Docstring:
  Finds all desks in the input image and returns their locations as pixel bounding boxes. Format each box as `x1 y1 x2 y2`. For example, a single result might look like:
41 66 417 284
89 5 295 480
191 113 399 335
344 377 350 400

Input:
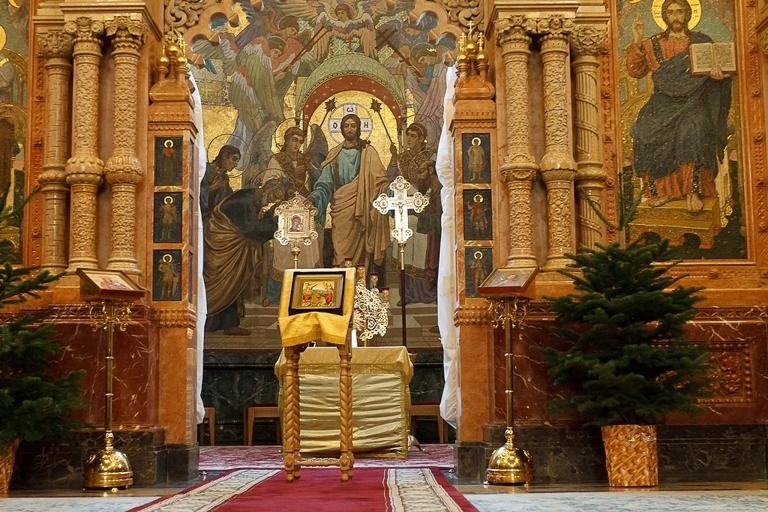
276 346 409 460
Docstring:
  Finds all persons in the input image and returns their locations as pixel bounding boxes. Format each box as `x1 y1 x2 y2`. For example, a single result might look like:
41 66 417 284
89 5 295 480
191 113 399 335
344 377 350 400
204 178 287 336
289 215 301 232
200 145 243 219
159 195 177 241
158 138 178 186
470 250 488 298
307 114 391 291
467 137 488 182
491 272 518 286
263 127 310 198
158 253 178 298
386 122 443 306
626 0 733 215
467 194 489 238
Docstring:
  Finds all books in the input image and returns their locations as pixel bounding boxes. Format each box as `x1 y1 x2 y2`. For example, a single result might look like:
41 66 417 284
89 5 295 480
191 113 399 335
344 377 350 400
688 42 738 76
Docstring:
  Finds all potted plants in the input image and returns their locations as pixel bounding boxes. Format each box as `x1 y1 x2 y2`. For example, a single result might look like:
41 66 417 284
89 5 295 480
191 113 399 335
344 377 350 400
0 180 85 498
535 172 715 487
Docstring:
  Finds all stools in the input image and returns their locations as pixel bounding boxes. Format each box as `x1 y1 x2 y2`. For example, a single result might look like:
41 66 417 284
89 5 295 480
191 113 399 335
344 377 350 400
198 402 449 447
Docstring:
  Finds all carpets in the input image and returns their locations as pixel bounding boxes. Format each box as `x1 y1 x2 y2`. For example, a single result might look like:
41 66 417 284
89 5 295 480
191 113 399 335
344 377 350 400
129 468 477 512
197 447 455 469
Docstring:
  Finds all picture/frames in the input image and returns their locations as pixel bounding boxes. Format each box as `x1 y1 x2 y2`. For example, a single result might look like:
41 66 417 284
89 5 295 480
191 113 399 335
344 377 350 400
77 267 147 298
479 266 537 294
288 271 346 315
